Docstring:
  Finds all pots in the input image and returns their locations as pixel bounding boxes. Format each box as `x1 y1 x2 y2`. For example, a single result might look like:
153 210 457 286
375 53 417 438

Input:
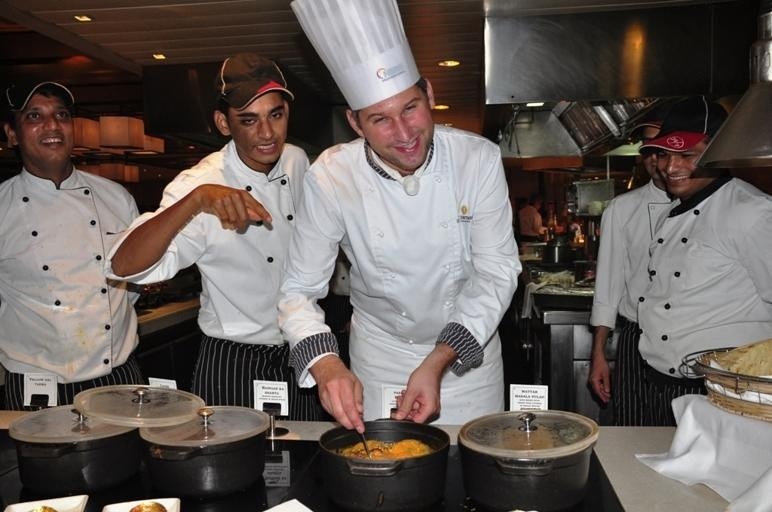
547 244 572 265
315 412 598 511
9 385 268 499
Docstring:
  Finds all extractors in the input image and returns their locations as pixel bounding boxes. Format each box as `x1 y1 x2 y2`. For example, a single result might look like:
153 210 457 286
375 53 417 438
483 36 695 158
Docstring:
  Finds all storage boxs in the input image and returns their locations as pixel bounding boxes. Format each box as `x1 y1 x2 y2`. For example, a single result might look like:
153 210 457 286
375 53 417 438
70 116 164 182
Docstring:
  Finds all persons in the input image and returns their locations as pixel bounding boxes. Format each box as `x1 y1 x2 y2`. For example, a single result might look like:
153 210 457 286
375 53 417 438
586 98 676 426
517 192 549 243
1 73 147 410
637 93 770 427
274 74 522 434
101 52 328 422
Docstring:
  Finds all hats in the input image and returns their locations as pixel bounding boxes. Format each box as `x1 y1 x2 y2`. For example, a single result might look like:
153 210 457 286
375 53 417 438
1 77 75 112
214 53 295 111
630 97 730 154
288 0 422 111
